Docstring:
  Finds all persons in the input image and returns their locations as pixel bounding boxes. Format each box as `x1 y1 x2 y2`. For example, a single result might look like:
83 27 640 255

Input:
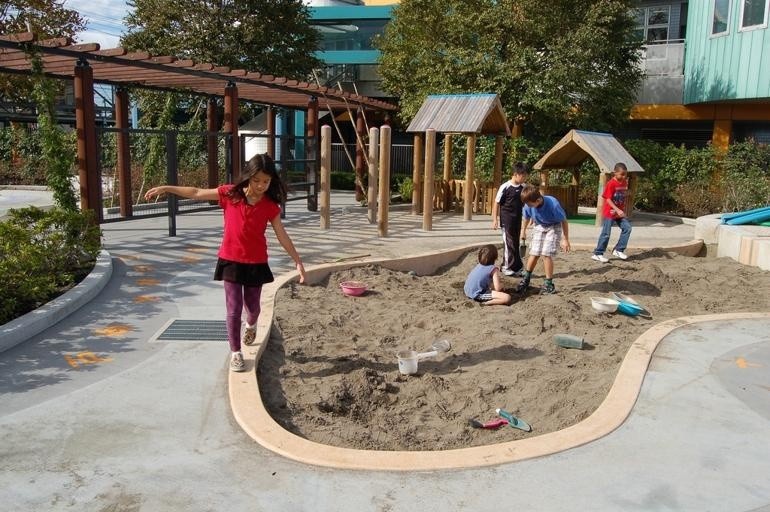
590 162 632 263
515 186 571 295
464 244 512 306
144 153 306 372
492 161 530 277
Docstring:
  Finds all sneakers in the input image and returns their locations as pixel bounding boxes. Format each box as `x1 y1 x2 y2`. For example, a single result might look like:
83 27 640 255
243 328 256 345
539 284 556 295
516 279 529 292
501 266 523 277
591 254 609 263
612 249 628 260
231 353 245 371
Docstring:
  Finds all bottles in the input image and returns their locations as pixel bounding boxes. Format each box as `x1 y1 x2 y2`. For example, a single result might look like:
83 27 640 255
553 334 585 350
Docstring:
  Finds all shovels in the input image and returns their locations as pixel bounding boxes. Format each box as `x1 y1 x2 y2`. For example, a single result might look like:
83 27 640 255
496 408 531 432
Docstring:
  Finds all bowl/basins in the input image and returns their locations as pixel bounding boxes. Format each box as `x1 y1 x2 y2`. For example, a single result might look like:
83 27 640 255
340 281 368 296
590 291 643 317
